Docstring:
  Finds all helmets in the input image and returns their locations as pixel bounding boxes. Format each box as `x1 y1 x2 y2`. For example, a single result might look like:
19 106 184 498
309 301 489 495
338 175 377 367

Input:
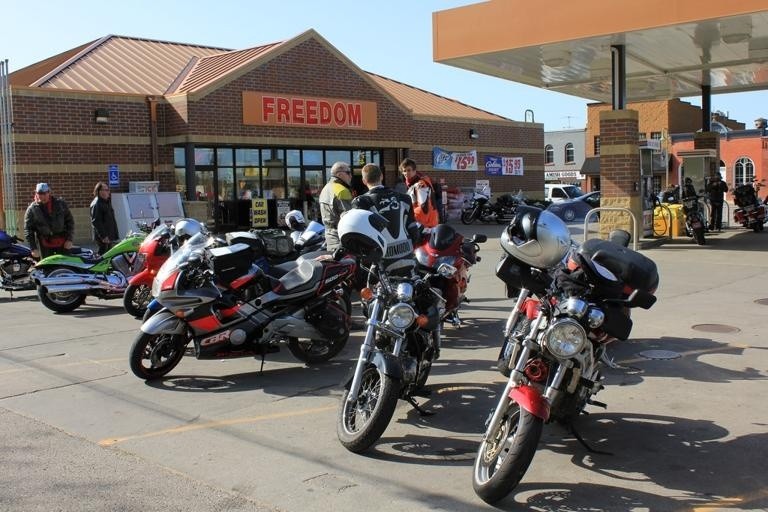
284 210 307 230
174 218 205 245
497 205 573 272
337 206 390 259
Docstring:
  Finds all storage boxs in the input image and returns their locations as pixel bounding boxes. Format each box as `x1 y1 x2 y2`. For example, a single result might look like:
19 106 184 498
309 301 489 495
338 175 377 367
577 237 660 307
733 208 745 224
204 242 253 287
494 249 551 296
757 203 768 220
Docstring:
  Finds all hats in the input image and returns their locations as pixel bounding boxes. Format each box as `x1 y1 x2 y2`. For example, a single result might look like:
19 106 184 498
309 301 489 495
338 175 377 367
33 182 50 194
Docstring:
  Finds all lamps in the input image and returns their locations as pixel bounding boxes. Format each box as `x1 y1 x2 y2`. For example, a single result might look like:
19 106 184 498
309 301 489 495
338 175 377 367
468 129 480 140
94 107 109 124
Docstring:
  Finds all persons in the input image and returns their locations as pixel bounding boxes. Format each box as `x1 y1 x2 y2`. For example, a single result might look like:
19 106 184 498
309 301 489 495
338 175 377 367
89 181 120 256
351 163 419 282
319 161 354 252
398 159 440 228
705 171 728 231
24 183 75 260
682 177 699 203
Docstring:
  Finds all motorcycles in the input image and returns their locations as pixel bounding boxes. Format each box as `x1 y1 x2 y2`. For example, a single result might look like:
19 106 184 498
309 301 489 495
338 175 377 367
461 183 553 226
667 182 710 246
727 175 768 233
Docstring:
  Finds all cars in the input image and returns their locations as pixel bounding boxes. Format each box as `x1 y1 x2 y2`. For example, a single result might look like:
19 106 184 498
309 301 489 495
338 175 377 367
549 190 600 222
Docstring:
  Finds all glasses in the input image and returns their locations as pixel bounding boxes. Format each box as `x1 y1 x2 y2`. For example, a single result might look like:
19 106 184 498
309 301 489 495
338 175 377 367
334 169 352 176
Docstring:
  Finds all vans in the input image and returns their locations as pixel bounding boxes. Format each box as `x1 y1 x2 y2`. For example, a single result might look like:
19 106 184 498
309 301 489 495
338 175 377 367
543 181 584 202
